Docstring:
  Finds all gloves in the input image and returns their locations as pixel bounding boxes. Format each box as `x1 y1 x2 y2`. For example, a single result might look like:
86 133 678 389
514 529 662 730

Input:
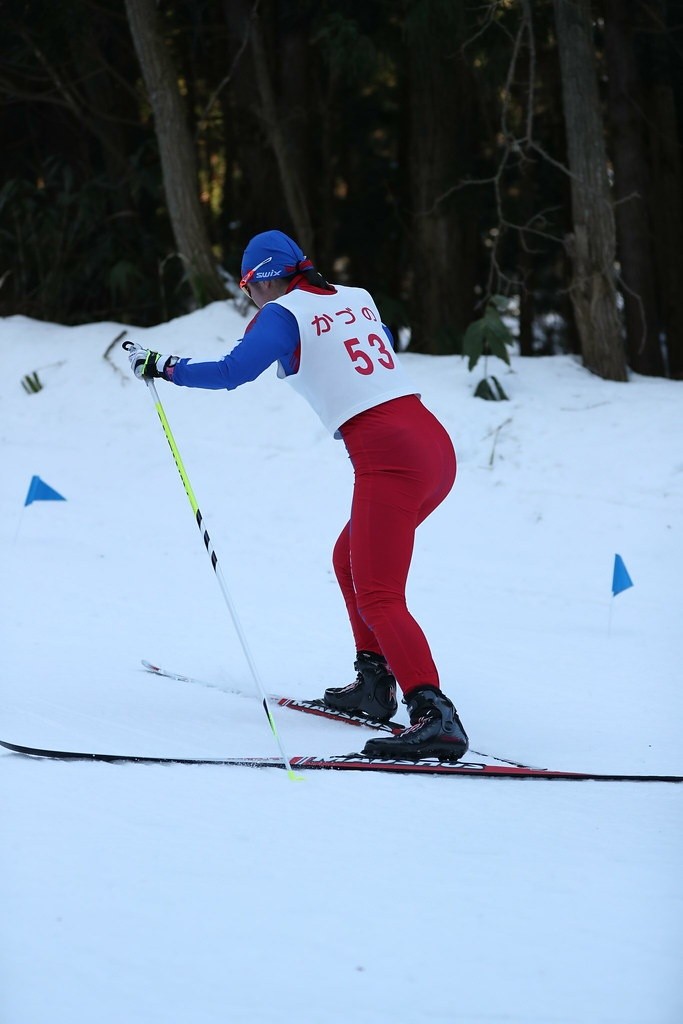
129 344 179 380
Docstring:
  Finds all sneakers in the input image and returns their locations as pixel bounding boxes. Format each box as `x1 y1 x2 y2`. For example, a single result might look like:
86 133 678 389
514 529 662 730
325 651 398 721
364 687 468 763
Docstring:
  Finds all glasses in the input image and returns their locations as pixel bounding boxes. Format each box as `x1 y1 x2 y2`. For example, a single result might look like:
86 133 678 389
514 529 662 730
240 257 273 299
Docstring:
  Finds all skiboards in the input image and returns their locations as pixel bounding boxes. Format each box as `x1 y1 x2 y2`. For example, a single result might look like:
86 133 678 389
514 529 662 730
0 653 683 790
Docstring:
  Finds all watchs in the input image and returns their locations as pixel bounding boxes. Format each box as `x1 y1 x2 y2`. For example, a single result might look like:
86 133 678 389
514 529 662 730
167 356 180 374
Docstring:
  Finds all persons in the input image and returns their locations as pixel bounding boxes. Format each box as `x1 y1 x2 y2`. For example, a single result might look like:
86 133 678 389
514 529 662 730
129 231 468 761
